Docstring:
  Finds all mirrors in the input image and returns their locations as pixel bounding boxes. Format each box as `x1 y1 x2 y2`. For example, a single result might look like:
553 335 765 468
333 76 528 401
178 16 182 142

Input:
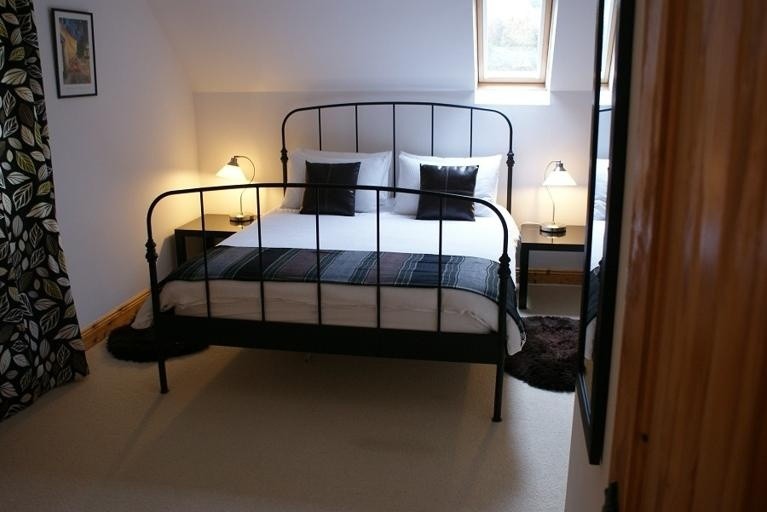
576 0 637 465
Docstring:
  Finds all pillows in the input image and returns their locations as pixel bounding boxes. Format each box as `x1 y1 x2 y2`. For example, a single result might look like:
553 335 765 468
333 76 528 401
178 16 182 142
279 147 503 220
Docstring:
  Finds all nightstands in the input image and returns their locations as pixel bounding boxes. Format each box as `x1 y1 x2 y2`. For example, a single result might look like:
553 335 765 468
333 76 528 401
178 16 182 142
519 224 586 309
175 213 257 267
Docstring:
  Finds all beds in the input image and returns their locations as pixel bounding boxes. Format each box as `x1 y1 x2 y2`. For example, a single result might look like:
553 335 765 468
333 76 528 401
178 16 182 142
146 102 526 424
582 107 613 409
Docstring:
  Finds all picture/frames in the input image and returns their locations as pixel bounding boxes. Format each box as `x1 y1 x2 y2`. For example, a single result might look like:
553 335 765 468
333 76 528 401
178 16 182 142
51 8 97 98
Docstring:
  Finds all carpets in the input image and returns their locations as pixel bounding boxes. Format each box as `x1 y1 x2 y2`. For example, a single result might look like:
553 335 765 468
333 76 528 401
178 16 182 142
505 315 580 394
108 326 208 362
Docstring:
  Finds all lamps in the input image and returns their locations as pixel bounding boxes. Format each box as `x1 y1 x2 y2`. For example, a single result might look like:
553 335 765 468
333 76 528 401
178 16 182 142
539 159 577 236
214 155 256 222
